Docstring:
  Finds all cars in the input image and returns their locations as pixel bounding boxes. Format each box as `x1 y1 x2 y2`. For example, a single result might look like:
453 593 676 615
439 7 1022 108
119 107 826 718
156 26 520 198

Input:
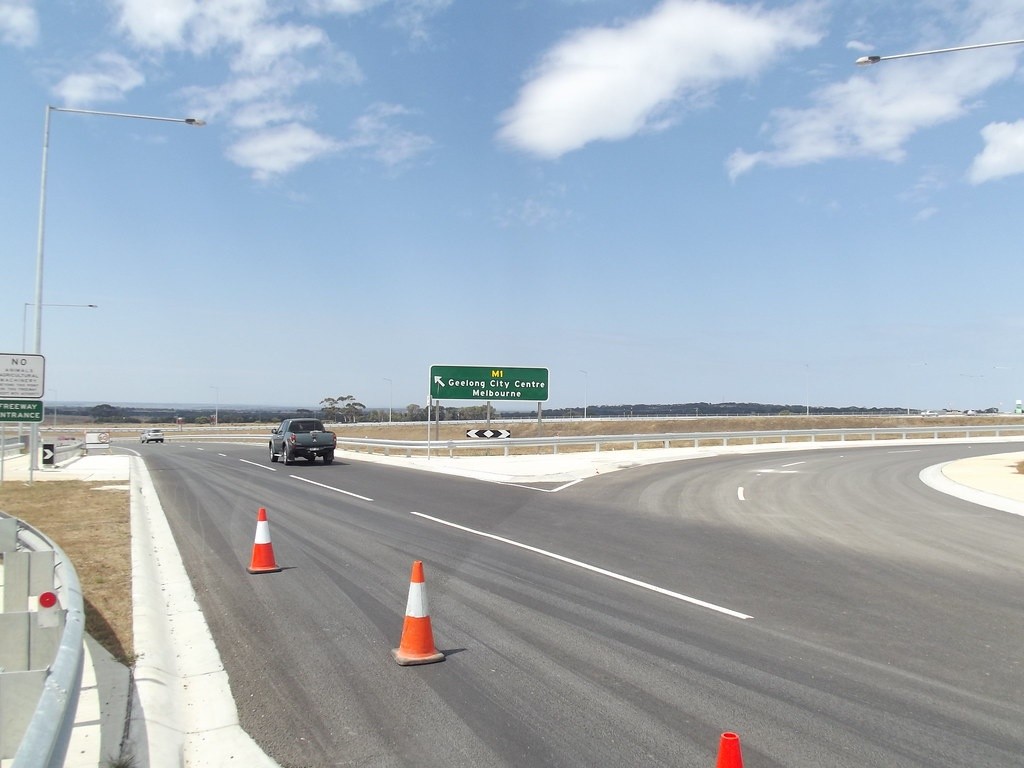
920 409 939 418
141 427 164 444
967 409 978 416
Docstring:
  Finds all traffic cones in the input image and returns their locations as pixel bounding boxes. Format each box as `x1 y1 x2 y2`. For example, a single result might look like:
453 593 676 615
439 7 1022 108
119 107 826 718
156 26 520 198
247 507 282 574
391 559 446 666
716 731 745 768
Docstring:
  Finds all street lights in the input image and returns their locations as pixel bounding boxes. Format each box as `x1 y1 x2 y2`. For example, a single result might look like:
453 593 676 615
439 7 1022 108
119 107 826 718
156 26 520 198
211 386 218 426
21 302 99 354
28 104 206 477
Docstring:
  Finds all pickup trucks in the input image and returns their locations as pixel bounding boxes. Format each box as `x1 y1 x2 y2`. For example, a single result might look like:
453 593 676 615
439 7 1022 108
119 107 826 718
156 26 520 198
268 417 338 466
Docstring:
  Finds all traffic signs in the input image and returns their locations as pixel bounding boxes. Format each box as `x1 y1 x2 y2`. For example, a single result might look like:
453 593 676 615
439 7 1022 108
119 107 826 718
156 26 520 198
428 364 550 402
